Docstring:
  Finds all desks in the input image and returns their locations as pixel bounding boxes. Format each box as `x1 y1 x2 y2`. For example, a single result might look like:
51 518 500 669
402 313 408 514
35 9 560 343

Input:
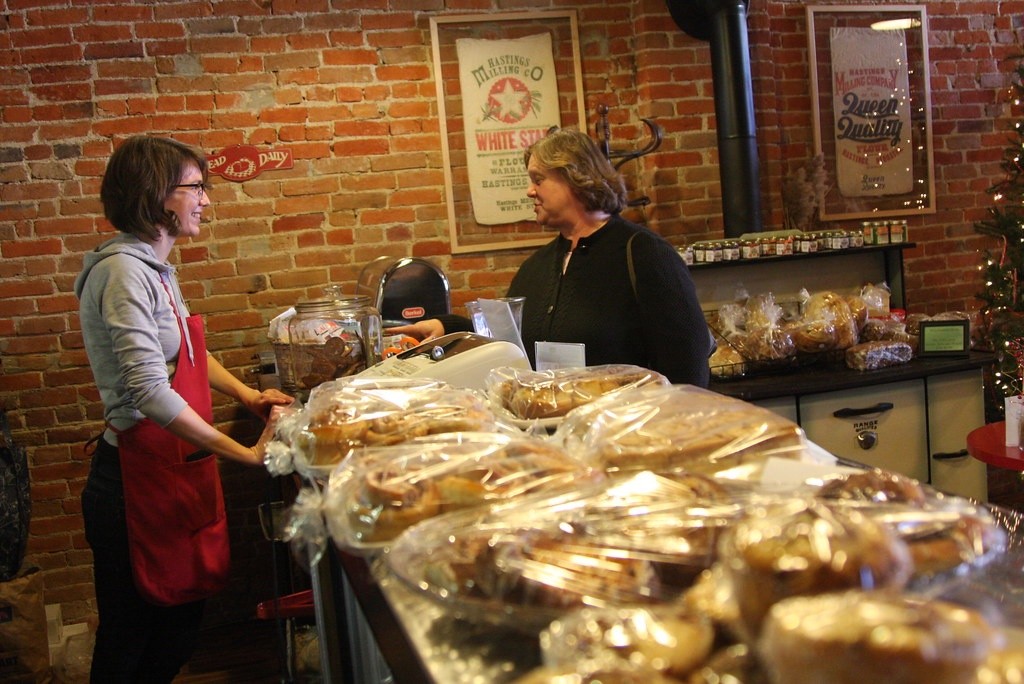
967 421 1024 472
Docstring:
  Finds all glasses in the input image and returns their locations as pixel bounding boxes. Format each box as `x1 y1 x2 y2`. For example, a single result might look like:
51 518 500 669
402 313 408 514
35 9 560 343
171 180 206 199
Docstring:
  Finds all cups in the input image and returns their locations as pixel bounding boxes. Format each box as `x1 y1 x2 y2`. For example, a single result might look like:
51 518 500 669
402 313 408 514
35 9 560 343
289 286 382 402
465 297 524 342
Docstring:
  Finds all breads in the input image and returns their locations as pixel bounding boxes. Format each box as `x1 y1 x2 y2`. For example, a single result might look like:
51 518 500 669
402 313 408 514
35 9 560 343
306 367 1024 684
707 290 986 370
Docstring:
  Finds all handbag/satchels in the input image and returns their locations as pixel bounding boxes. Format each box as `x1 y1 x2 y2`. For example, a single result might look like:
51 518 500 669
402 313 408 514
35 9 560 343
626 231 717 383
0 407 31 581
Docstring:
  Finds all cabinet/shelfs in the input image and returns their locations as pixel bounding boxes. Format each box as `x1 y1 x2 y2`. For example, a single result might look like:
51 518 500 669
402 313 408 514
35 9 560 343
684 242 998 505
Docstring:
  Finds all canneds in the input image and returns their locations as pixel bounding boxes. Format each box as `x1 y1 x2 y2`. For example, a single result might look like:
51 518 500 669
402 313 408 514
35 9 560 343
675 220 908 265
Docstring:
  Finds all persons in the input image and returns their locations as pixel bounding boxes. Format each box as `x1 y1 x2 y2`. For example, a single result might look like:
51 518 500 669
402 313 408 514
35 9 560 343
74 133 297 684
383 128 711 391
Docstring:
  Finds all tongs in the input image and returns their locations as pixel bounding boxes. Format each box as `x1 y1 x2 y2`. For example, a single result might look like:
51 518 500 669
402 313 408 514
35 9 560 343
256 590 314 619
